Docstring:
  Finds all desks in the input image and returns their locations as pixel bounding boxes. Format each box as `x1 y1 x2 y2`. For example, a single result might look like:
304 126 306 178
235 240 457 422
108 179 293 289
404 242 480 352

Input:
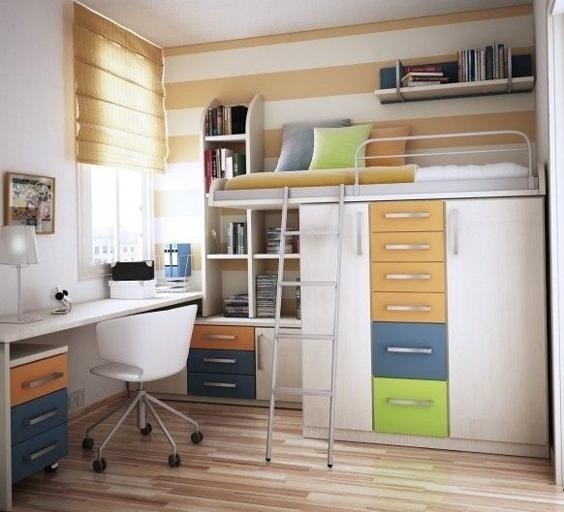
0 289 202 512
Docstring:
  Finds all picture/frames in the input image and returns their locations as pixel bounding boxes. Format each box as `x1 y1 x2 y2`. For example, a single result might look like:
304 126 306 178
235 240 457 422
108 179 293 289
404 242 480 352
4 172 55 234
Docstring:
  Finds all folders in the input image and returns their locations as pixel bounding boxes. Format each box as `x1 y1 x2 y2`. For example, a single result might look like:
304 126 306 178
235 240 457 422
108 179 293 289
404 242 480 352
164 243 192 279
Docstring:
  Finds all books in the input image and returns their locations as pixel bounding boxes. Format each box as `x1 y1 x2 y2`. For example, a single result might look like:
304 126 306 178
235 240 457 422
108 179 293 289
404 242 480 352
223 222 301 319
205 104 245 194
401 39 513 86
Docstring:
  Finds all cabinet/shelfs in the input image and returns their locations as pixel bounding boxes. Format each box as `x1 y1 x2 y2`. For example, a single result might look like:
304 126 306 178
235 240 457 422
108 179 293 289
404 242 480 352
127 365 187 396
201 93 264 197
8 343 71 488
186 323 255 404
202 207 300 323
254 327 302 409
297 194 551 459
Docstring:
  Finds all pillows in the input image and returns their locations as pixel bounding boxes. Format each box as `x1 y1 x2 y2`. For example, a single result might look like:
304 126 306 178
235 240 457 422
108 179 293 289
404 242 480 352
365 126 411 167
308 123 374 170
275 118 351 173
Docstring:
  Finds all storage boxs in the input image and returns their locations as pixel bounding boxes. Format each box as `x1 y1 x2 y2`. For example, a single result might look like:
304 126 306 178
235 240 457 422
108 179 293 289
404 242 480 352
108 258 156 281
108 279 157 300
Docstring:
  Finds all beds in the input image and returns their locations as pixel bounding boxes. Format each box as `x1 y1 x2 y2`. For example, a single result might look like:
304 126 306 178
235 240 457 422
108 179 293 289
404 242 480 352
205 123 546 207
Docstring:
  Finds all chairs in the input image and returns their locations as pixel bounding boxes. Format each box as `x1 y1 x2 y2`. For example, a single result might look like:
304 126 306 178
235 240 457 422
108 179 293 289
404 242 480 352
79 302 204 471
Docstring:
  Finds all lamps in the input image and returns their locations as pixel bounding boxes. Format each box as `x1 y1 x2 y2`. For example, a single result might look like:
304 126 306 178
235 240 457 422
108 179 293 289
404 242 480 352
0 224 43 324
